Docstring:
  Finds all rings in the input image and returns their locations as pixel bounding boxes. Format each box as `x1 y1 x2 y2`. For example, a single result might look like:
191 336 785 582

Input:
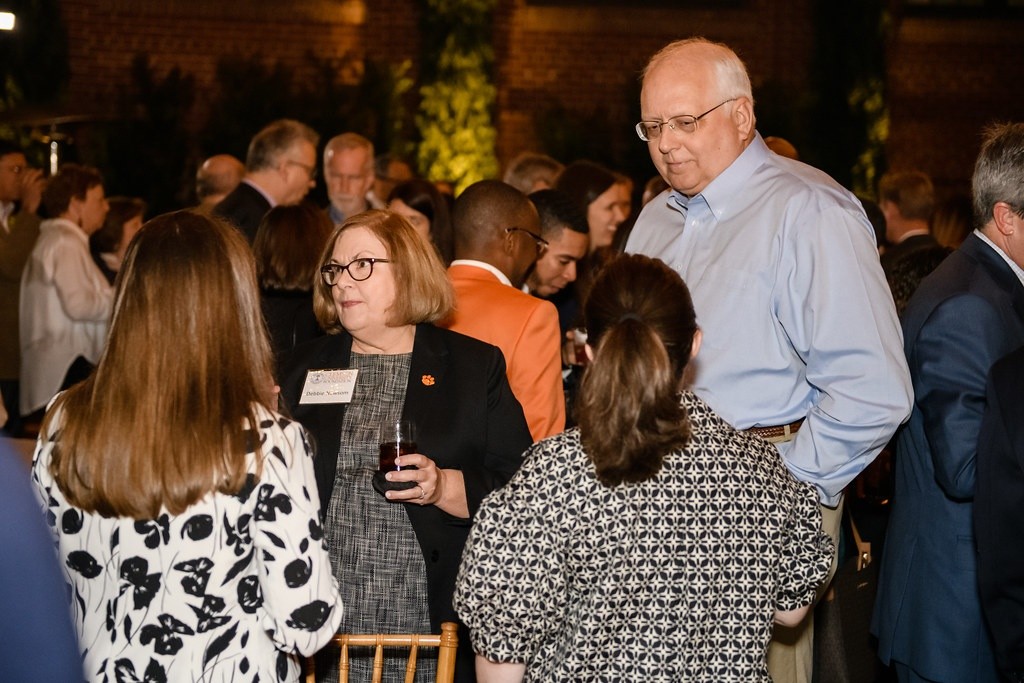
418 485 424 499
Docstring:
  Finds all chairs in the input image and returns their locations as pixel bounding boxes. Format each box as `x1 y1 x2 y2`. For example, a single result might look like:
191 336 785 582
306 622 459 683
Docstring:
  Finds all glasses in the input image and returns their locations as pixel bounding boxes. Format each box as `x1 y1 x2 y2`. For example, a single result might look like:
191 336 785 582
320 257 397 285
505 228 550 258
288 160 318 181
2 164 25 174
635 99 741 142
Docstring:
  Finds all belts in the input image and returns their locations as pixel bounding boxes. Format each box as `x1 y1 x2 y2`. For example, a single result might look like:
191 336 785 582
742 416 806 438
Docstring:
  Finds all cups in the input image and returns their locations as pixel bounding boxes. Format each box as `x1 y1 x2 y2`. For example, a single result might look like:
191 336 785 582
379 419 419 472
573 326 588 364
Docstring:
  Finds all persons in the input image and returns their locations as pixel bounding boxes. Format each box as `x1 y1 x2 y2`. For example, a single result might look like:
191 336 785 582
270 208 535 683
870 121 1024 683
197 154 246 214
250 202 335 385
452 253 835 683
29 209 343 683
19 163 118 420
213 119 320 245
98 195 157 277
877 170 956 304
623 39 915 683
0 137 47 439
387 177 448 246
503 152 671 366
323 132 376 225
433 179 567 443
